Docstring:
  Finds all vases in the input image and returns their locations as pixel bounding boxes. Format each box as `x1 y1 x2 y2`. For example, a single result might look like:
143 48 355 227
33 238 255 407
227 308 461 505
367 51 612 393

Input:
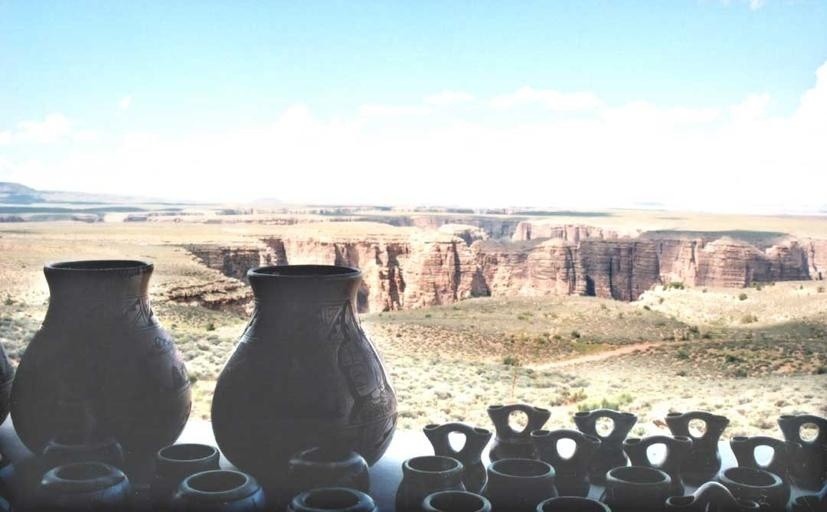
8 256 195 473
210 263 397 479
